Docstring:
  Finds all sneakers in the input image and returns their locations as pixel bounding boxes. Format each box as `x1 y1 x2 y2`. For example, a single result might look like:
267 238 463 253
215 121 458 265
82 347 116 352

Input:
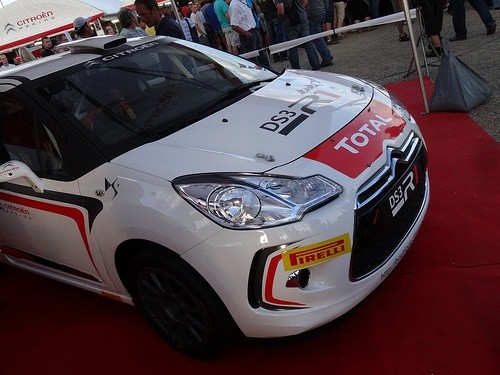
272 54 290 62
325 40 339 45
427 39 443 50
310 64 321 71
487 21 497 35
426 48 443 58
448 32 467 42
320 59 333 67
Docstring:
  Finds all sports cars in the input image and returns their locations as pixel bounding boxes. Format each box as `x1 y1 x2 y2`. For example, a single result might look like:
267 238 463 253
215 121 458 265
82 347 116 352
0 33 431 362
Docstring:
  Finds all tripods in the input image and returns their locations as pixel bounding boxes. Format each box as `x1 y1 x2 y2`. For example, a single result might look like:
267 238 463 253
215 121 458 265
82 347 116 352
400 0 440 80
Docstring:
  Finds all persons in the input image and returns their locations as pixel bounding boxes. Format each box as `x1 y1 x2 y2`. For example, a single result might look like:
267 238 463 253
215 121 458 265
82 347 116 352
256 0 289 63
117 8 148 38
53 33 71 53
228 0 270 70
305 0 334 67
244 0 394 56
175 10 197 42
186 0 228 64
449 0 496 41
391 0 410 42
134 0 196 79
443 0 500 15
0 53 16 73
70 17 94 40
18 46 37 64
137 17 147 31
160 0 205 19
418 0 444 57
274 0 322 70
214 0 242 55
105 26 115 35
41 36 56 58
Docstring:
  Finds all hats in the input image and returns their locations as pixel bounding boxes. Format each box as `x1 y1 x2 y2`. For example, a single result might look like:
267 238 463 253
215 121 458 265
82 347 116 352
72 17 92 35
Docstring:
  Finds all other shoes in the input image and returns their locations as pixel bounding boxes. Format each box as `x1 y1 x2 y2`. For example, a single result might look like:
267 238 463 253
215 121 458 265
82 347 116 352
336 34 345 41
355 27 376 34
399 32 409 42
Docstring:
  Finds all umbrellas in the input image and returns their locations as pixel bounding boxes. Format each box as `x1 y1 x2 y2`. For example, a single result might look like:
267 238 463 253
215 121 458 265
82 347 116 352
0 0 108 55
